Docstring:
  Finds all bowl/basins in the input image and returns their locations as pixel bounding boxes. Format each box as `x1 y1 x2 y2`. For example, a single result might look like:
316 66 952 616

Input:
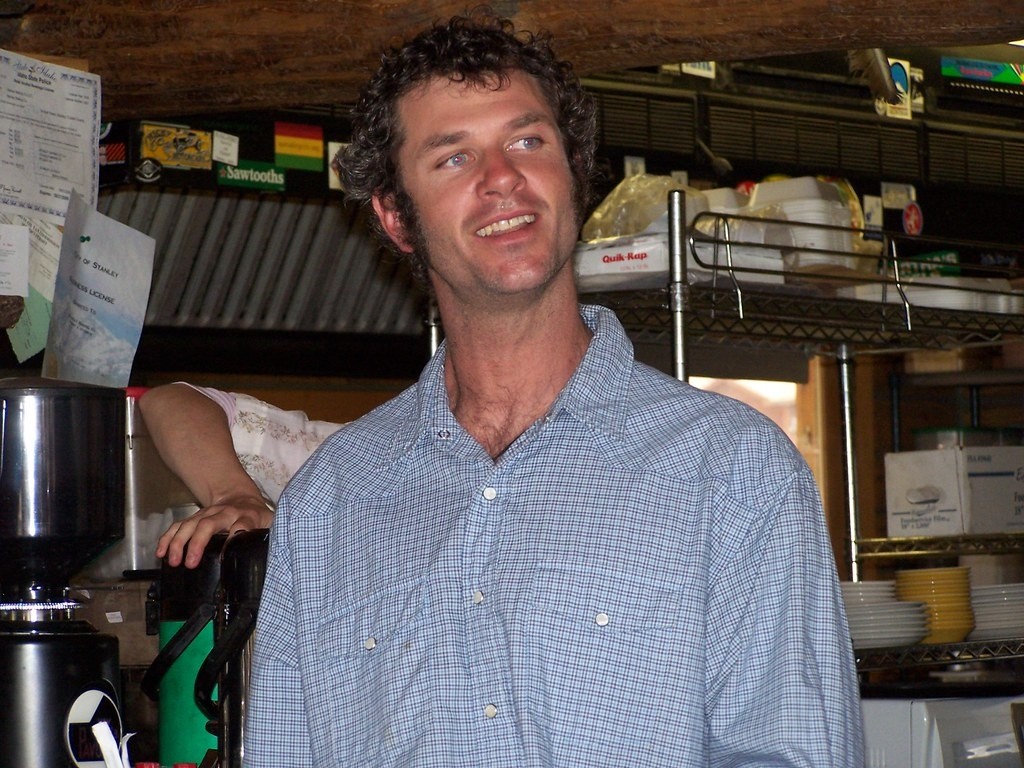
643 176 860 275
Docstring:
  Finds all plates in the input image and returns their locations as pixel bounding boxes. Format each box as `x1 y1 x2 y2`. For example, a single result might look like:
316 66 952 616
839 553 1024 650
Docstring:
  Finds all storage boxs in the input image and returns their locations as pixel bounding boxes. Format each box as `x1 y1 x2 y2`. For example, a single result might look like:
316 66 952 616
884 447 1024 538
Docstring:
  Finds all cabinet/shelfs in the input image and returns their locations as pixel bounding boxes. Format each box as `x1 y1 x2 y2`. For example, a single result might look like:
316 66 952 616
571 190 1024 768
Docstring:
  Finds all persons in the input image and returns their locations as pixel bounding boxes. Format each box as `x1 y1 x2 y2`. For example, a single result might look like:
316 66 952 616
242 16 866 768
138 382 350 570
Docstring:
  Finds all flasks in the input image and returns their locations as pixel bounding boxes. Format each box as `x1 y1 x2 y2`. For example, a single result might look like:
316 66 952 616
153 527 268 768
